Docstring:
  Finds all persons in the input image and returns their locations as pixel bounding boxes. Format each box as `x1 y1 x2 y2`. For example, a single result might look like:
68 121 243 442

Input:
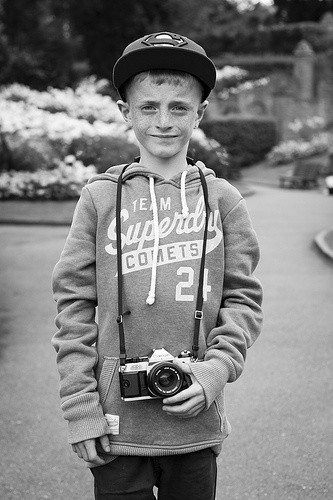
52 32 263 500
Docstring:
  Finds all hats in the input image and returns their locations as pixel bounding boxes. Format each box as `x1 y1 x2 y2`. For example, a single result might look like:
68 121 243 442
113 32 217 102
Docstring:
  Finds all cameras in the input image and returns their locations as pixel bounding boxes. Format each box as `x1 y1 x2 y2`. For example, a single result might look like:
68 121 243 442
119 348 193 402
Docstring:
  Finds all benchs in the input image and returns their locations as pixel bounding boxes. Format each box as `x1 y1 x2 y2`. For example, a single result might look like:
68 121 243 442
279 164 323 189
